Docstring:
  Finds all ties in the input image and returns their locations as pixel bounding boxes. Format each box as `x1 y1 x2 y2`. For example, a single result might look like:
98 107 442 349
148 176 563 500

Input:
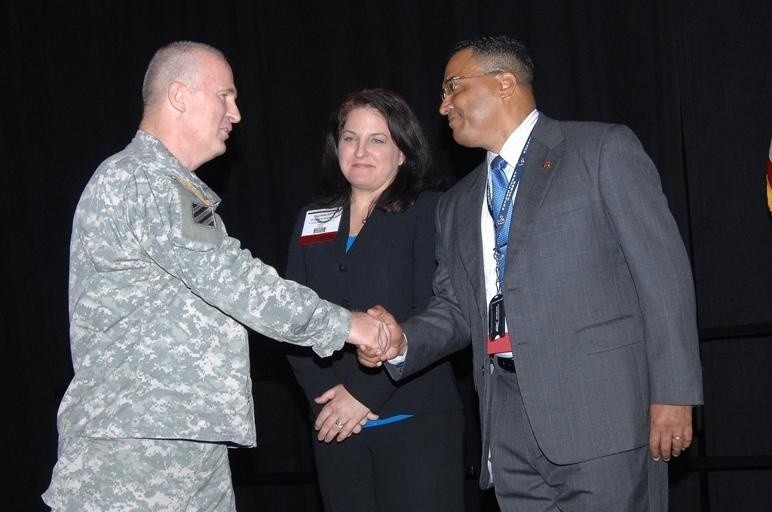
489 156 513 284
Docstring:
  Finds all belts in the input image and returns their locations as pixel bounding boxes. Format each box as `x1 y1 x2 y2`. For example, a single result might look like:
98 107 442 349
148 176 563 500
498 356 516 372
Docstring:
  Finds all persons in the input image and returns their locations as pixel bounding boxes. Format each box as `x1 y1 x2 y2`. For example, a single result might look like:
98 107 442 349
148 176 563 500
40 36 389 512
355 28 706 512
278 86 474 511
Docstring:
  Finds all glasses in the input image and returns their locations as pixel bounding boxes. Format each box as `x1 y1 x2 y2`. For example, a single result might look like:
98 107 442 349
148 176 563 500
440 74 484 101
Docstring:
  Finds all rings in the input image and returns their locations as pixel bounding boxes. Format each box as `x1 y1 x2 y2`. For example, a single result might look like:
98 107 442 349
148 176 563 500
671 435 683 440
334 419 343 428
